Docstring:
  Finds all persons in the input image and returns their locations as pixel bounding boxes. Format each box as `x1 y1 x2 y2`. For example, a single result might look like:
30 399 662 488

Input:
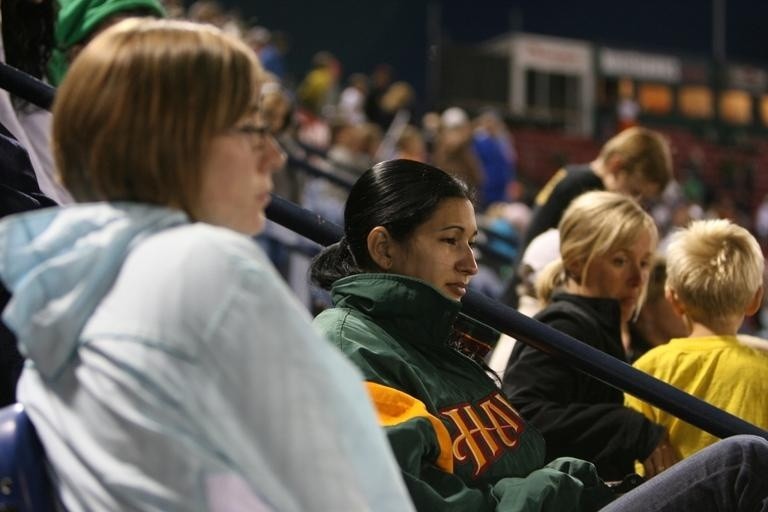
618 215 768 460
502 191 695 485
0 1 767 396
295 156 768 509
0 14 420 510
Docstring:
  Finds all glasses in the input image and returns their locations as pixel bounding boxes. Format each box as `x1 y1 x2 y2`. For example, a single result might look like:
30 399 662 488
220 124 269 171
